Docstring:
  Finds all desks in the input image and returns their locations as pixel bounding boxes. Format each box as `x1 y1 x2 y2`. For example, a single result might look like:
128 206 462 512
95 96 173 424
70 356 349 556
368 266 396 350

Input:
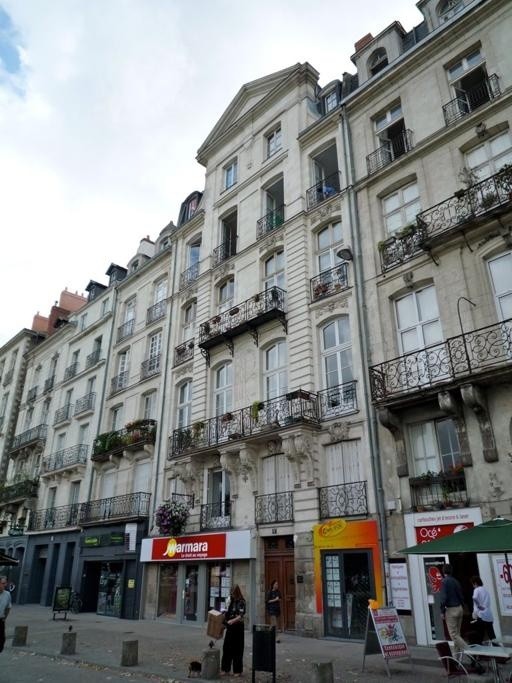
464 644 511 682
492 633 512 646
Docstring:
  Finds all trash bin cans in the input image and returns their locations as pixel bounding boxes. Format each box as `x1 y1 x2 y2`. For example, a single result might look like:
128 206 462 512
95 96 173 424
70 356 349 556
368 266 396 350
252 624 276 683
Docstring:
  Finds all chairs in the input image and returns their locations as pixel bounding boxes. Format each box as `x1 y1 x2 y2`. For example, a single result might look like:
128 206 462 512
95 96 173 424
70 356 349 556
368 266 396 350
436 641 485 682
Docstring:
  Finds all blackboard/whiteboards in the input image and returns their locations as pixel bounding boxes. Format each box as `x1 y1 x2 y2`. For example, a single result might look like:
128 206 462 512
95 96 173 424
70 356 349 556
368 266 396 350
52 586 72 611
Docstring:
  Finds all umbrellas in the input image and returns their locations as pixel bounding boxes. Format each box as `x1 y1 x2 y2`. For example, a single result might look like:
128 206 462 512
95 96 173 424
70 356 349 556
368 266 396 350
397 516 512 594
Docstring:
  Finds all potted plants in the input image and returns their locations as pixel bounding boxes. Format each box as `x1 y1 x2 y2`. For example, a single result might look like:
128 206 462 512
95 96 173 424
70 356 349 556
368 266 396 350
409 462 467 512
178 390 312 454
94 417 154 456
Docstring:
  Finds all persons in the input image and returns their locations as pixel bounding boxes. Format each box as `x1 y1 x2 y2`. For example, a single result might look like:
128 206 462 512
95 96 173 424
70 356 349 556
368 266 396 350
264 579 282 644
439 564 485 677
0 576 12 653
219 584 246 677
467 576 505 668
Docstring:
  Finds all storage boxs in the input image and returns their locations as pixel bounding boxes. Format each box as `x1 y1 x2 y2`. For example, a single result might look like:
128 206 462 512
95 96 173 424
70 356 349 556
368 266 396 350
206 608 225 638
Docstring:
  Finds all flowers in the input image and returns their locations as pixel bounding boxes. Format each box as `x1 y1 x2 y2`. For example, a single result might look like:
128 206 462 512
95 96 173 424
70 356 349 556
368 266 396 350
153 496 191 537
